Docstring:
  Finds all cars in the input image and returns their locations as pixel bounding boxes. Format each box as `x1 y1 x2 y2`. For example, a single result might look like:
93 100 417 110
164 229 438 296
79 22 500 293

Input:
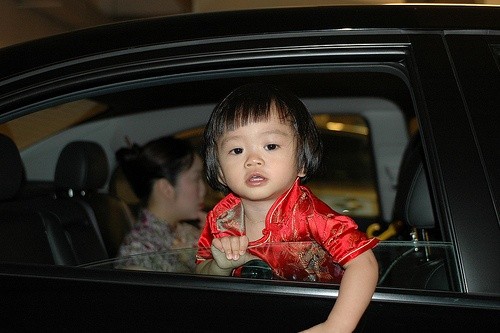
1 3 499 333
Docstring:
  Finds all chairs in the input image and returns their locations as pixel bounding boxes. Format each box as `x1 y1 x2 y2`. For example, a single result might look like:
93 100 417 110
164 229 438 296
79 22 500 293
42 137 140 272
1 130 67 265
368 123 455 293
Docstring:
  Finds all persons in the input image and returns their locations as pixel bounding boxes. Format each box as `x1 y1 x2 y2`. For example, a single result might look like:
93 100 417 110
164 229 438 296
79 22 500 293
111 135 209 274
193 79 380 333
